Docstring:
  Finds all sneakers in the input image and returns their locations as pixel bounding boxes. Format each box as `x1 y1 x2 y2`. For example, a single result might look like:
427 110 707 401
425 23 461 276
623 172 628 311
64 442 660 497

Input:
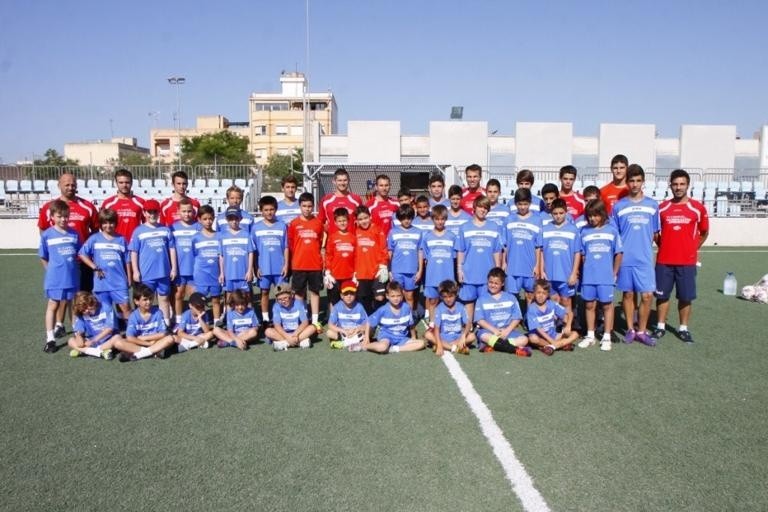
652 326 669 342
623 327 634 344
637 328 658 348
54 323 66 337
422 322 576 357
599 333 614 351
578 330 596 353
674 328 695 345
38 340 56 356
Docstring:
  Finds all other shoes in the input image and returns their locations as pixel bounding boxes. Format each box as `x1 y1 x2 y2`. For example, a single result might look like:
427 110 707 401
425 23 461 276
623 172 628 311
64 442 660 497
330 341 344 349
102 349 112 360
297 338 311 348
70 349 84 357
349 343 364 352
119 352 137 362
156 349 165 358
199 341 209 349
314 324 322 332
232 339 247 350
218 340 229 347
273 340 290 351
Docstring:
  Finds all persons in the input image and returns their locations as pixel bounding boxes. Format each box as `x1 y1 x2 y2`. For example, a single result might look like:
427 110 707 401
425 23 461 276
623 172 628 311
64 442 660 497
38 155 709 363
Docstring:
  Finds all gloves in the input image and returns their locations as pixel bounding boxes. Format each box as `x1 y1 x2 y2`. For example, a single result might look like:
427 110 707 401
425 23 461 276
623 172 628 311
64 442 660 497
351 270 360 286
321 269 337 292
374 263 389 284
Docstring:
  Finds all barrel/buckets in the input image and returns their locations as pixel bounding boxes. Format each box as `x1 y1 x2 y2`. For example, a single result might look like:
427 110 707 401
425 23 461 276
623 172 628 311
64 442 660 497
723 272 737 296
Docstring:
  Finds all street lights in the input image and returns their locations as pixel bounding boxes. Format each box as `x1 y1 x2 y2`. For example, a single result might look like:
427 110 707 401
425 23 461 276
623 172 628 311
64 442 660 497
148 110 161 129
167 77 187 175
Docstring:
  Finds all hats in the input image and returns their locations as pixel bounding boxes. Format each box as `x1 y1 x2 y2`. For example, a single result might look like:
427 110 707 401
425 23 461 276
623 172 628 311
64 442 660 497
340 280 357 293
224 205 242 218
273 282 296 297
189 291 209 306
143 199 162 213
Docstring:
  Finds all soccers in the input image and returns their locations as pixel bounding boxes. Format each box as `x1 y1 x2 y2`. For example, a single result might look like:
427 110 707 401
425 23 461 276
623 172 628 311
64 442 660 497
741 274 768 303
